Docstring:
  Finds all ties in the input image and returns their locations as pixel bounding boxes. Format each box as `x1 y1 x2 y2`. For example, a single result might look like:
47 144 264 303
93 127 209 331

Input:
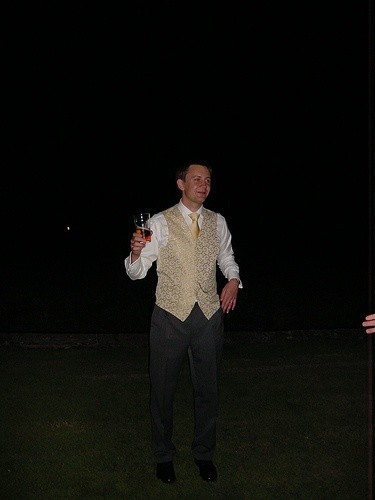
188 212 201 238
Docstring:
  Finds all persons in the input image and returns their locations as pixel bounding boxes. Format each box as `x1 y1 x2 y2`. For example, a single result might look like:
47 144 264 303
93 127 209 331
124 159 243 483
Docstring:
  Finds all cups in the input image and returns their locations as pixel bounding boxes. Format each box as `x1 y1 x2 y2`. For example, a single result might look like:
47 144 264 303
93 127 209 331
135 212 152 242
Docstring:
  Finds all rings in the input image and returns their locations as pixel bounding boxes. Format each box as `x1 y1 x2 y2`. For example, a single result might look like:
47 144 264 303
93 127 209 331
229 301 233 303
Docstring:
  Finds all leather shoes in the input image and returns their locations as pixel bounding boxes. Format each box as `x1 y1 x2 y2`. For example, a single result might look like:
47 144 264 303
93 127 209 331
156 464 176 484
194 457 217 482
